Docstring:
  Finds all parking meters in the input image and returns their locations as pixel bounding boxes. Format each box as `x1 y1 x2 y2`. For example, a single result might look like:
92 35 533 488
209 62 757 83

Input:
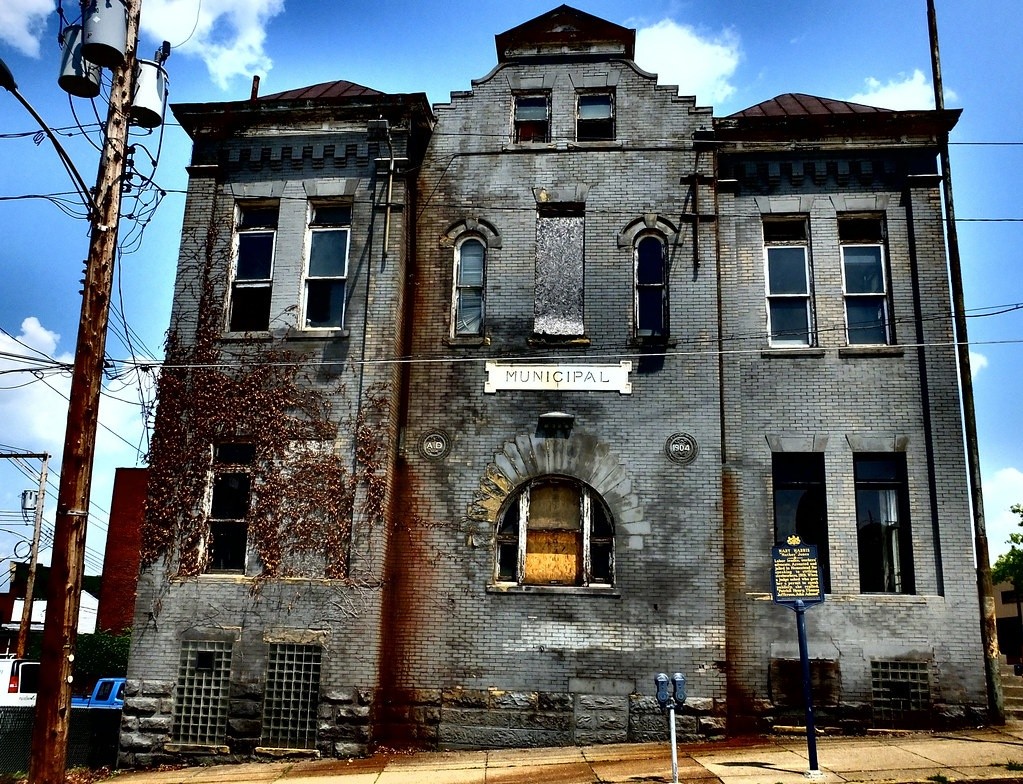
654 672 687 784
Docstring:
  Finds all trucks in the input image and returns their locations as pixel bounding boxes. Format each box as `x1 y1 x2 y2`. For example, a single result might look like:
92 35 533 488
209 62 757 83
71 678 126 708
0 659 42 709
